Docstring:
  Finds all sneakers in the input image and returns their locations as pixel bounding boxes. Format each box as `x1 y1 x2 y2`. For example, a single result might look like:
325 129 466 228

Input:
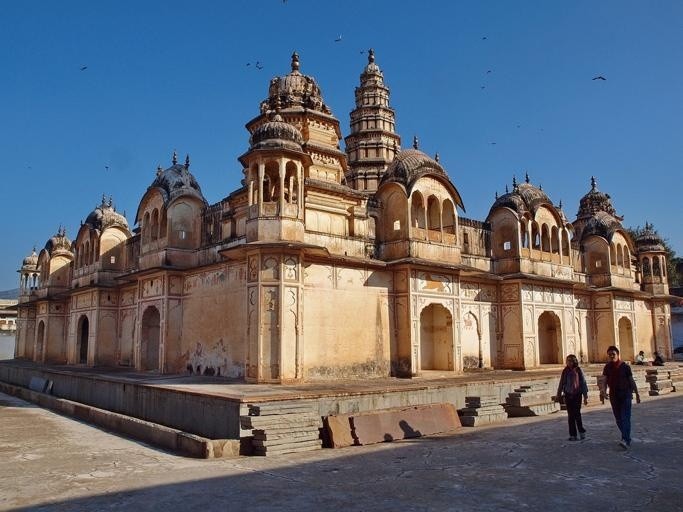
568 433 631 449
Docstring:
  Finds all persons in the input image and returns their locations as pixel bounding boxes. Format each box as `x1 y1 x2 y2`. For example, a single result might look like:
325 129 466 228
598 346 640 450
633 350 645 365
554 355 588 441
652 351 665 366
